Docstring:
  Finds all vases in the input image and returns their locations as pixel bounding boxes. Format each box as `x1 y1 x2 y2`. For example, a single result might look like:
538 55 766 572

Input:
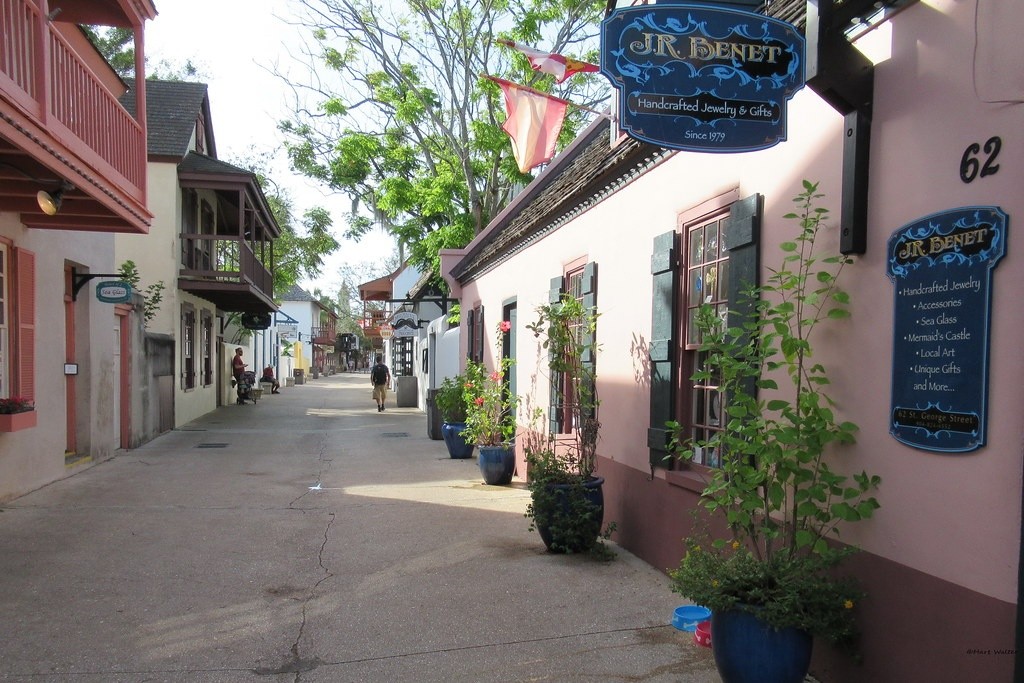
476 441 517 486
705 599 819 683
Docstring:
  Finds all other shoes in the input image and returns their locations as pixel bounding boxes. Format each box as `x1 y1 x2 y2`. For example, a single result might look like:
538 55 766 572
378 405 385 412
271 388 280 394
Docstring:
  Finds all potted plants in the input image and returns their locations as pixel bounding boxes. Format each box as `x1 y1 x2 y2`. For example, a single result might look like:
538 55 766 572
523 291 615 561
435 358 489 460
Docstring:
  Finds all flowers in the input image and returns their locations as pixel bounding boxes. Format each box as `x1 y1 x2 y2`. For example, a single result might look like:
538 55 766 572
666 538 868 639
458 318 544 449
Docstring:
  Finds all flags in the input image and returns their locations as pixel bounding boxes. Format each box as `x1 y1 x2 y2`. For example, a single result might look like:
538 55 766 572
507 41 599 85
487 76 569 175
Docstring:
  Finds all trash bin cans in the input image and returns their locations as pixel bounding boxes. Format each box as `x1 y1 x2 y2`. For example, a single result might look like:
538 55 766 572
394 375 418 408
293 368 304 385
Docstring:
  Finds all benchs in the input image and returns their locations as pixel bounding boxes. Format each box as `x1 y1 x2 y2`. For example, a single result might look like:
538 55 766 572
260 379 274 395
285 376 296 387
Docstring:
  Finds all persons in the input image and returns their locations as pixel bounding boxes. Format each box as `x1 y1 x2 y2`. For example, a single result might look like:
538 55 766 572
263 364 281 394
231 347 248 405
370 356 391 411
